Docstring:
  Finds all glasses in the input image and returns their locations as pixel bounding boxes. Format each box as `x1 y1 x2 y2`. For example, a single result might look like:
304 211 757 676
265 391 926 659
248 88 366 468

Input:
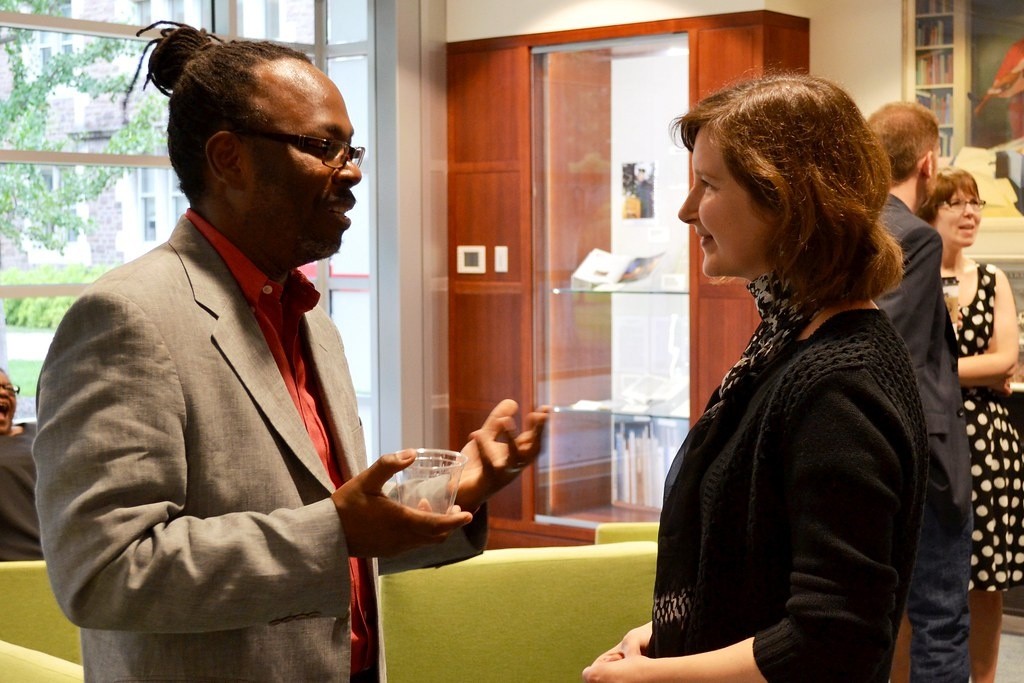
934 198 986 212
0 382 20 393
222 124 366 171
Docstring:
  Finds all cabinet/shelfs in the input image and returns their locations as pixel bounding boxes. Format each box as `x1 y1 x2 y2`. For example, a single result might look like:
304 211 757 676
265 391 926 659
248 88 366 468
900 0 972 169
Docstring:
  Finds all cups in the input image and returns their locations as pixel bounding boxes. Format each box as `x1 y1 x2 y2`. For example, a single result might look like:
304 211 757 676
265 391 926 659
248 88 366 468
394 444 466 516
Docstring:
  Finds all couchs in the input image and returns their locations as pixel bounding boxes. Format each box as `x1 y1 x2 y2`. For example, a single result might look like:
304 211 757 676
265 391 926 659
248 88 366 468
0 516 663 683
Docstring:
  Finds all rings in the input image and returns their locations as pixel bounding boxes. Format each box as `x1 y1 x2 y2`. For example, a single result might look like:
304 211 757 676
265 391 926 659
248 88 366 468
510 462 526 475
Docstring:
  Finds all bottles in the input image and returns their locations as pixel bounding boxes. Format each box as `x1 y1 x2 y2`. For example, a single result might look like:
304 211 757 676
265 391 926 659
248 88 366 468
938 276 960 338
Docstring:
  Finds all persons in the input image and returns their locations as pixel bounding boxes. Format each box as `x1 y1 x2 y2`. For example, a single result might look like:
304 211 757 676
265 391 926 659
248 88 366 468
29 24 554 683
869 101 972 682
582 75 928 683
0 368 38 564
885 169 1023 683
